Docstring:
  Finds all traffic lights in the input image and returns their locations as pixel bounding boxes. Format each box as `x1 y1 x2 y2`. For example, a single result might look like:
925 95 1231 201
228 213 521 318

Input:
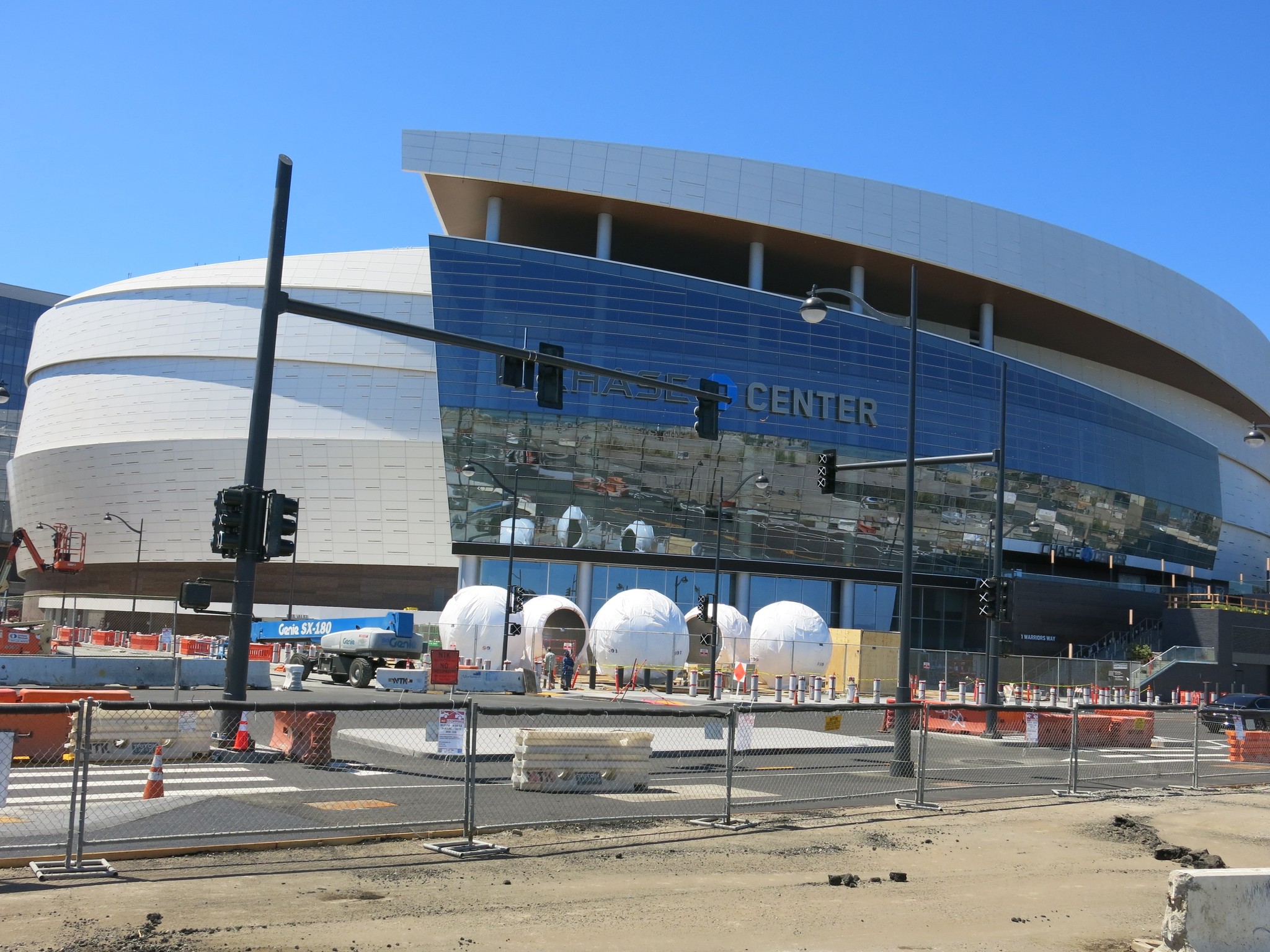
693 378 721 440
511 624 522 634
265 492 300 557
697 595 709 621
700 634 710 645
817 449 835 494
979 578 998 618
217 488 242 552
513 587 524 613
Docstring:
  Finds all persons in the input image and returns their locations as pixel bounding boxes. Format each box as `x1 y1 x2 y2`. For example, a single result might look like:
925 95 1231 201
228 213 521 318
542 646 555 689
562 650 575 690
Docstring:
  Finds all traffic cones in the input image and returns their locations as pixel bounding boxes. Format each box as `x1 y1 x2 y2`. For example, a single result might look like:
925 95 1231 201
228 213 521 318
231 710 256 753
141 746 165 800
851 687 862 704
791 690 799 706
876 708 891 733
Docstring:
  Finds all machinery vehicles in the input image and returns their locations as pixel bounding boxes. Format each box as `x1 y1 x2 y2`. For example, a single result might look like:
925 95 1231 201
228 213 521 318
251 609 429 690
0 521 87 654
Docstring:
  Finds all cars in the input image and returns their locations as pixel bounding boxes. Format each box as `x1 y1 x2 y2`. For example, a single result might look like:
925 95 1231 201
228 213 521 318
1200 694 1270 735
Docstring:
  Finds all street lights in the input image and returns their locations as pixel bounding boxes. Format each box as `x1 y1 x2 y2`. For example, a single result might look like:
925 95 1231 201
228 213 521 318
461 455 519 668
36 521 73 626
103 511 144 646
705 468 769 701
985 517 1042 707
798 263 921 780
